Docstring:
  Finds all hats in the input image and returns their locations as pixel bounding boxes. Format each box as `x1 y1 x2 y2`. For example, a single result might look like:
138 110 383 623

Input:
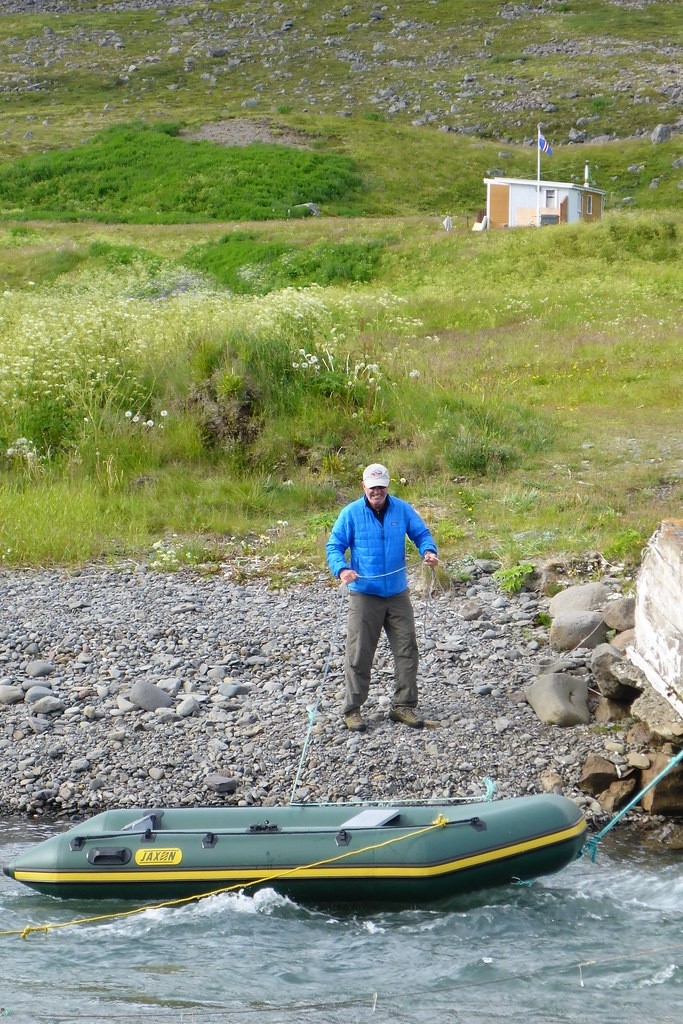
363 463 389 488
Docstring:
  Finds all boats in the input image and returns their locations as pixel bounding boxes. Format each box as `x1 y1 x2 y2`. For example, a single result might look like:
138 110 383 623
1 794 587 920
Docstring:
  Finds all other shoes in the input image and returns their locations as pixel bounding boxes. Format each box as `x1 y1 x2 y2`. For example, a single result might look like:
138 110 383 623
390 707 424 727
345 707 367 731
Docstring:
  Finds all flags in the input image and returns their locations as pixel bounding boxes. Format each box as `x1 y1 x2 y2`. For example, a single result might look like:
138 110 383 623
540 131 553 155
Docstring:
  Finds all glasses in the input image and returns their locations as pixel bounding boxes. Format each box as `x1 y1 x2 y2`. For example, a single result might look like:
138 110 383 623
369 486 387 489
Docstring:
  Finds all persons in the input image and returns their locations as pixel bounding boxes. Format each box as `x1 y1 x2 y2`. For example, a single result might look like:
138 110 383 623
326 464 437 731
